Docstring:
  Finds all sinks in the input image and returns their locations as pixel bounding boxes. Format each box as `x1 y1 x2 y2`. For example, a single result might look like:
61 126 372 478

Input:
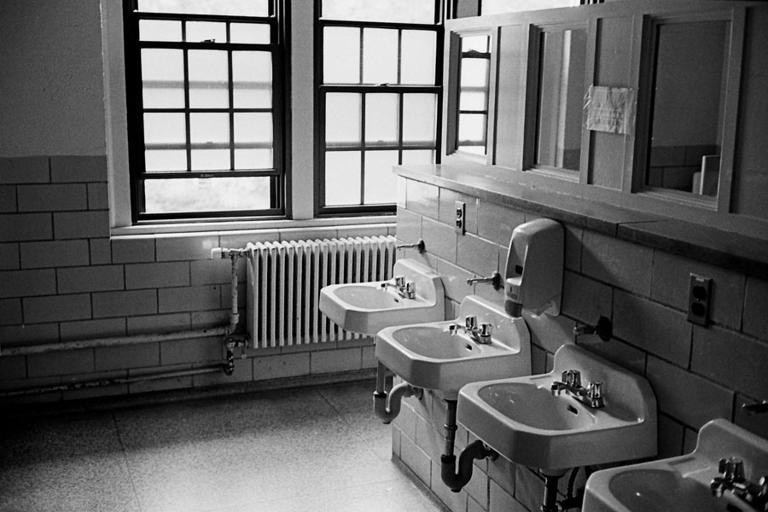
580 419 768 511
456 346 658 468
318 257 446 334
376 297 531 390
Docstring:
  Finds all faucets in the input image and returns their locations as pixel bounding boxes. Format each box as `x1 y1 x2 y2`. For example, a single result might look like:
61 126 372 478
710 455 767 512
550 369 605 410
448 315 493 343
380 275 417 299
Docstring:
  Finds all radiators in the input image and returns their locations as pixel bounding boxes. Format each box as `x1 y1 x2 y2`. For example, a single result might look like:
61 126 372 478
245 234 396 349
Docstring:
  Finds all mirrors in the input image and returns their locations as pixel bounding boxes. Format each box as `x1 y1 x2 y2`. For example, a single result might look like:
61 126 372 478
519 17 598 185
621 7 744 215
446 27 499 166
